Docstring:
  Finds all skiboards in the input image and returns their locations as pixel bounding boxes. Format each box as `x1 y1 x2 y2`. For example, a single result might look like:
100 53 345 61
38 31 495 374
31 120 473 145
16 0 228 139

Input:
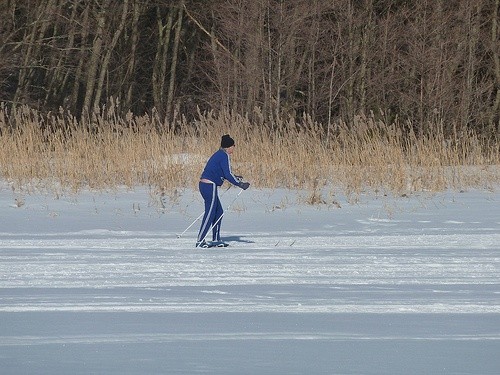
273 239 296 247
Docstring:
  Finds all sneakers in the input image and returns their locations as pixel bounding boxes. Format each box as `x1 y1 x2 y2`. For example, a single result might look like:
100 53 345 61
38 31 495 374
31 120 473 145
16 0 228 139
213 242 229 247
196 242 212 247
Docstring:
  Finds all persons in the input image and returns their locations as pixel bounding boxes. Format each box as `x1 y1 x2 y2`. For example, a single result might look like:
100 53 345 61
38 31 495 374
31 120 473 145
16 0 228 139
196 134 250 248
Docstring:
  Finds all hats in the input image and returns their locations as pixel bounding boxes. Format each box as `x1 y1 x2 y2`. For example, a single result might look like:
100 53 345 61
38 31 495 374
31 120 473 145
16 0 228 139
220 134 234 148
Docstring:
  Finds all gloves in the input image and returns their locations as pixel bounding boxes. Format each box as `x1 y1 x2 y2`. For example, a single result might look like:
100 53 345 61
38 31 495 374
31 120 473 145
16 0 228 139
239 182 250 190
235 176 243 180
